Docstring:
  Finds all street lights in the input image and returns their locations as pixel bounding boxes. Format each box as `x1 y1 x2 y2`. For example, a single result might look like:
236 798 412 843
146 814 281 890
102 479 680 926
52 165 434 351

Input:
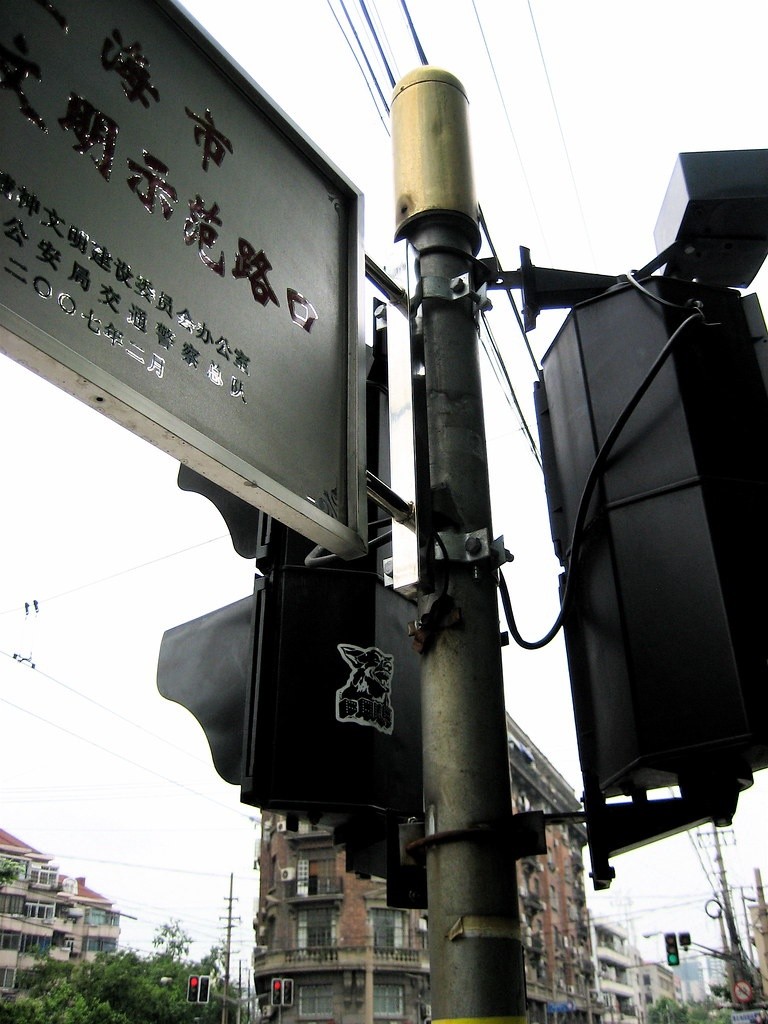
642 931 751 1012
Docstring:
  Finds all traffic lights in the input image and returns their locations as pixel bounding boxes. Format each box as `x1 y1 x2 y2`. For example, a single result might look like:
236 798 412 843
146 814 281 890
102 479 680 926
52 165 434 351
156 310 392 814
197 975 211 1004
282 979 295 1006
186 976 200 1003
271 978 283 1007
663 932 680 966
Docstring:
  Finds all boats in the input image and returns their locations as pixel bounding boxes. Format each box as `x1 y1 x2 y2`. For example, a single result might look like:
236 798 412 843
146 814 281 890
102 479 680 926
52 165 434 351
679 932 690 946
